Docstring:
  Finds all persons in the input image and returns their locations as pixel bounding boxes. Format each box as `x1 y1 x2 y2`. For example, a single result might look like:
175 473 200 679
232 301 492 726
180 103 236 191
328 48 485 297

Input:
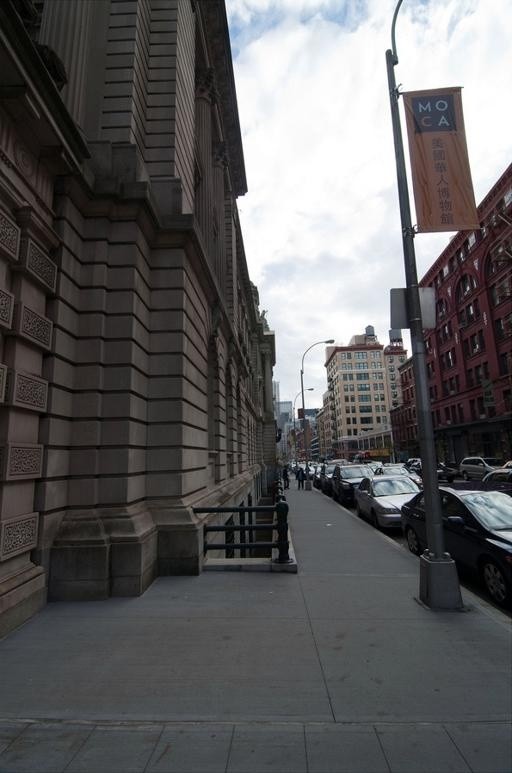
283 464 291 489
296 467 306 490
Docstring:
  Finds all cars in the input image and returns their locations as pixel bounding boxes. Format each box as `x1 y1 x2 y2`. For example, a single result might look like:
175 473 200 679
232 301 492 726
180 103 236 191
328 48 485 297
290 455 511 609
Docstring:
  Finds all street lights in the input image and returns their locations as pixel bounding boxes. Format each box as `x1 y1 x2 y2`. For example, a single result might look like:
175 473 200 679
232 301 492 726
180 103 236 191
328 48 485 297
293 340 334 480
